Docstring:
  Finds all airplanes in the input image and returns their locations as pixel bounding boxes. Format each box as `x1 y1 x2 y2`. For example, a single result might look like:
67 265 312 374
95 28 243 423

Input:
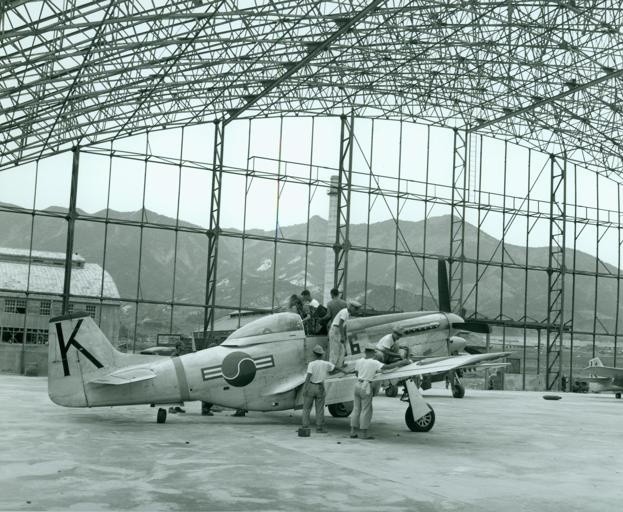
47 310 517 433
577 356 623 399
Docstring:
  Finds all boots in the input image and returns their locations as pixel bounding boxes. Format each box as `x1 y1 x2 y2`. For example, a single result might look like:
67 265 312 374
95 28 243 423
360 430 374 439
350 428 358 438
317 425 328 433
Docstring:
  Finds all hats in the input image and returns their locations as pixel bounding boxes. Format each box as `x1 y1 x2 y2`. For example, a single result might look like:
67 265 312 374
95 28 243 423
350 301 361 309
393 326 403 335
365 343 376 353
313 344 326 354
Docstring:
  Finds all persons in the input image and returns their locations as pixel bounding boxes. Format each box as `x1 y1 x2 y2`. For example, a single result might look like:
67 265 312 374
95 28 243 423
301 343 355 432
200 342 218 416
290 288 361 372
373 327 409 364
167 340 185 414
348 344 412 440
230 408 248 417
